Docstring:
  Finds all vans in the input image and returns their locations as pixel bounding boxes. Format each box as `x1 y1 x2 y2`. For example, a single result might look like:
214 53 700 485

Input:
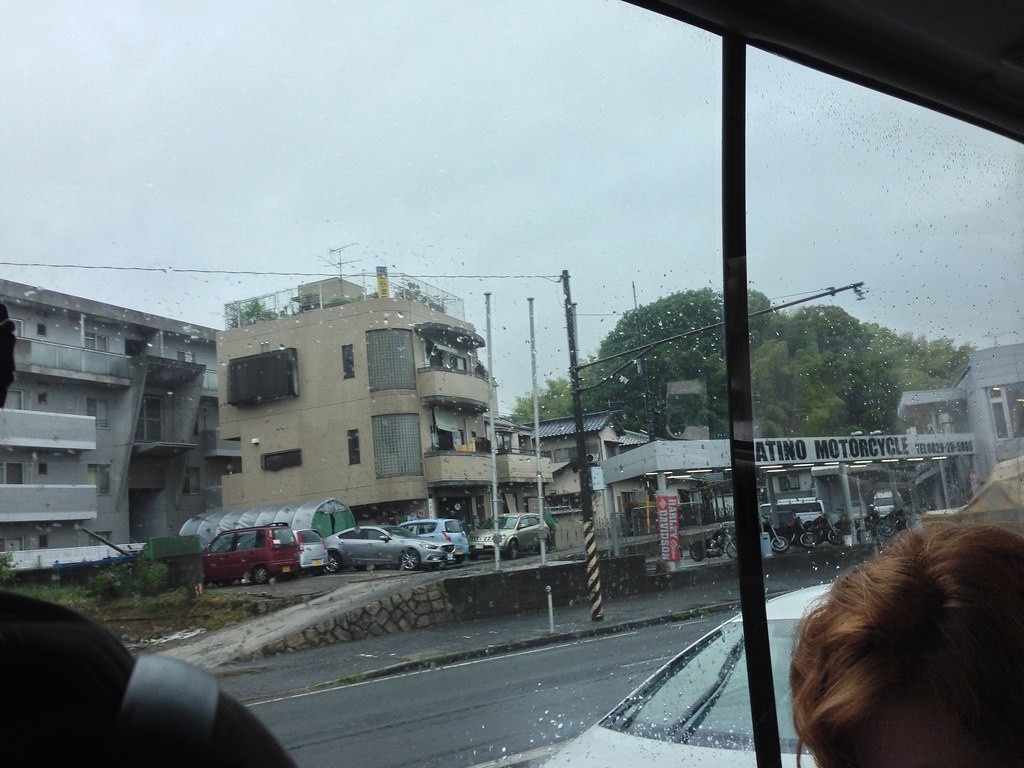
870 489 906 518
761 496 825 526
198 524 301 584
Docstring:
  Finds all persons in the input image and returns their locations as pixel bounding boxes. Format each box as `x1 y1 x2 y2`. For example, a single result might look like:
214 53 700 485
789 521 1024 768
0 298 300 766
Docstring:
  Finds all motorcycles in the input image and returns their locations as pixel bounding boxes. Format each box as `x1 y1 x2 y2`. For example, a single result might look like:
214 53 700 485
688 522 738 562
761 505 917 553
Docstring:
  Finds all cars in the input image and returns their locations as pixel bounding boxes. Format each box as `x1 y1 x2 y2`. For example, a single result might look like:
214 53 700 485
466 511 552 560
397 518 471 564
531 582 838 768
239 528 329 579
324 524 457 573
